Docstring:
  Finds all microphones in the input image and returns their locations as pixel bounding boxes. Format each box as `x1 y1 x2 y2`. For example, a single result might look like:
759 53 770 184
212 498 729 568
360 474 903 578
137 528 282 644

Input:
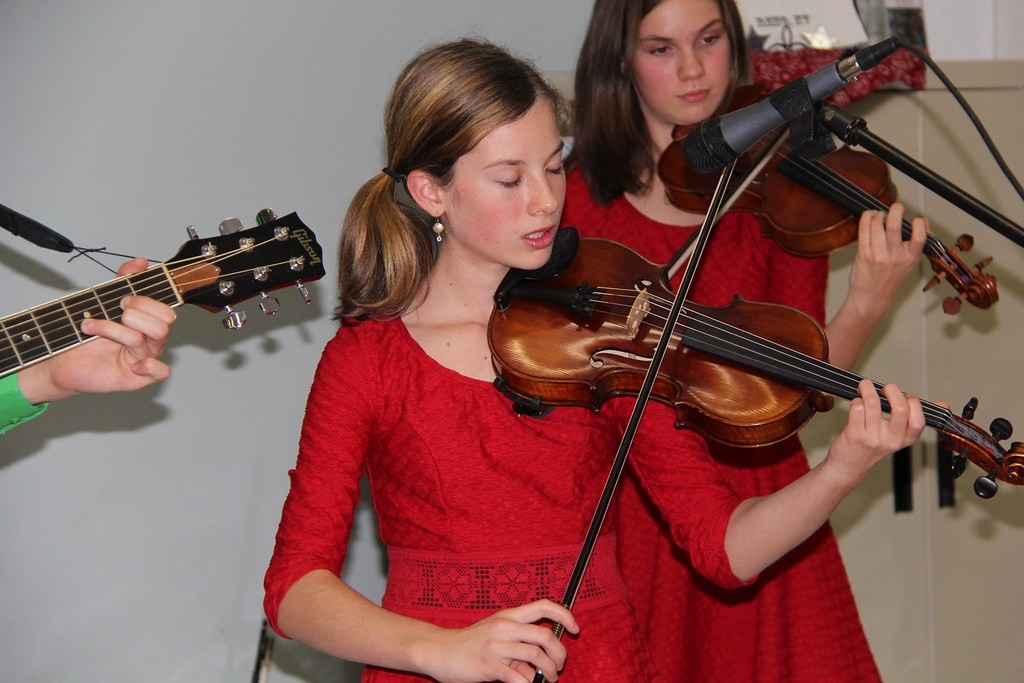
684 39 899 173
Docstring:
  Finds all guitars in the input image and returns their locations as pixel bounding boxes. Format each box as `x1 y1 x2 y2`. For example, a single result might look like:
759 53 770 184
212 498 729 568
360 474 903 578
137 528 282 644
0 204 329 385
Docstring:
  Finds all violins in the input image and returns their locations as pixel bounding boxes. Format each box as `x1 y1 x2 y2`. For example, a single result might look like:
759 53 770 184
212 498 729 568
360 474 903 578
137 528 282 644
485 224 1024 501
657 77 1002 317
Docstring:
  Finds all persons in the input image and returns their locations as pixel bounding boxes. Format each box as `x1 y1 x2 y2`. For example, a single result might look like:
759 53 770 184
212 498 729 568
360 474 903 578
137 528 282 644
258 40 927 683
562 0 930 683
2 255 179 437
